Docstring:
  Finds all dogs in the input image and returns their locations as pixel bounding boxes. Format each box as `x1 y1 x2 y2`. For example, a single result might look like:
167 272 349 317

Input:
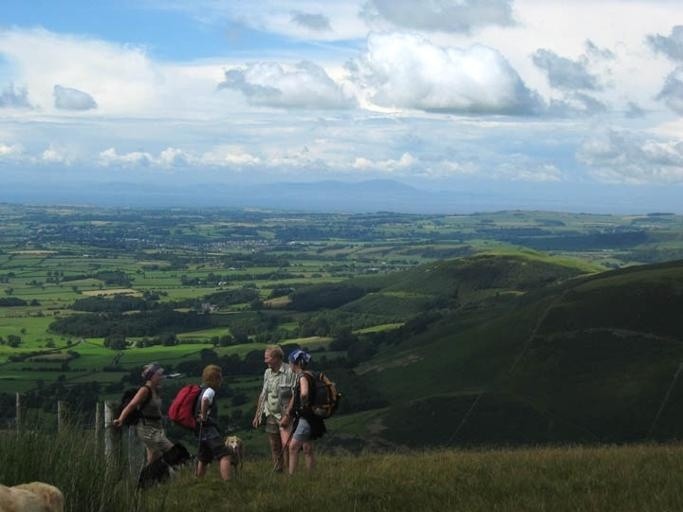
224 435 245 473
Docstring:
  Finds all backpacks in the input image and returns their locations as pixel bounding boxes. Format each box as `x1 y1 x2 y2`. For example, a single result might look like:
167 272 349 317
291 370 341 418
120 385 152 427
169 384 204 430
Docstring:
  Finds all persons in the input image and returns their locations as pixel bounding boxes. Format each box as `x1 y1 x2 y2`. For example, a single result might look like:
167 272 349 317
253 347 298 473
192 364 236 481
287 348 327 474
113 363 175 464
